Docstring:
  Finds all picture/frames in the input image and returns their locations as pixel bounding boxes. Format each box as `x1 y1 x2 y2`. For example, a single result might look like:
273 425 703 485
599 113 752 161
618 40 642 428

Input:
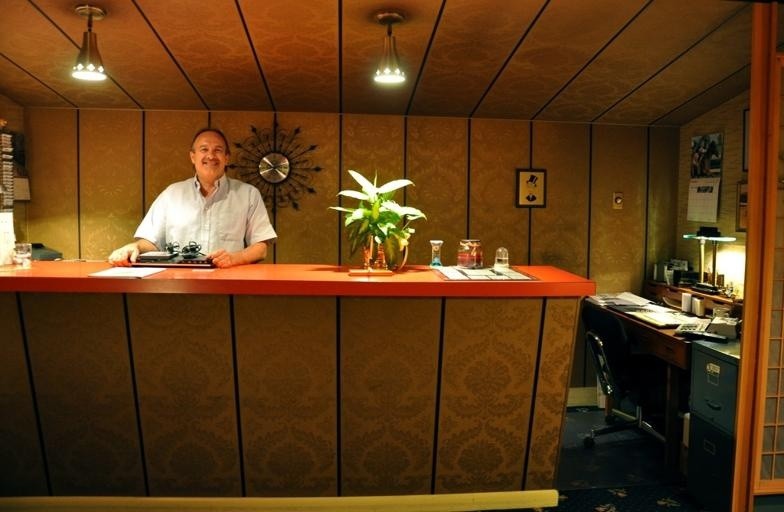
515 168 547 209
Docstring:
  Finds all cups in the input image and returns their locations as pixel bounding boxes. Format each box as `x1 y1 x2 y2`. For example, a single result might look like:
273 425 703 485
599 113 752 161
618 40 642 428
713 307 731 319
10 243 31 269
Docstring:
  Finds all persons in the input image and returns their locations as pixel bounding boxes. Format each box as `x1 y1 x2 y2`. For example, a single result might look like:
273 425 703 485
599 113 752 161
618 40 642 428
108 129 278 268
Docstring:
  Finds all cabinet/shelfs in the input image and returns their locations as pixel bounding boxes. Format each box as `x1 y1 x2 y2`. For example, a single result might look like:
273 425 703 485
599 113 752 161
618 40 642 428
685 338 741 511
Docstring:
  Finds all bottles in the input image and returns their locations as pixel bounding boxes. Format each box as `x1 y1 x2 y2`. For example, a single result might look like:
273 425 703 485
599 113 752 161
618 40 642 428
456 239 483 269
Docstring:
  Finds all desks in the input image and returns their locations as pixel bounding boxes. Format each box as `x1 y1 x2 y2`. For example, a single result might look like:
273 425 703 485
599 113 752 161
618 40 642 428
582 296 692 487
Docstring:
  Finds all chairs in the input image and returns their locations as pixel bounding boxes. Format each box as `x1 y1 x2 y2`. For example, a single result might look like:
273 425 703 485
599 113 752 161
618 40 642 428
582 304 668 468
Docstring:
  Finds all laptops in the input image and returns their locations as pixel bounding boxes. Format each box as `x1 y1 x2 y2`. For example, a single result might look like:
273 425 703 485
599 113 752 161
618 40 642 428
128 255 217 268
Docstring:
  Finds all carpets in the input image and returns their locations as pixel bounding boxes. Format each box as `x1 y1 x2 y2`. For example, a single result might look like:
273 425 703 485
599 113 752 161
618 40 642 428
542 471 706 512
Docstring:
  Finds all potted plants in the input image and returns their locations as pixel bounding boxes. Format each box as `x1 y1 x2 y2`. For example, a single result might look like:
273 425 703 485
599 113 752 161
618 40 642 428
328 167 428 272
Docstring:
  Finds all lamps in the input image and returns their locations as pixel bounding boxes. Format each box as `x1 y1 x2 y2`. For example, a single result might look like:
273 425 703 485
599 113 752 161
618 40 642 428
371 8 408 85
681 226 737 296
70 3 110 83
